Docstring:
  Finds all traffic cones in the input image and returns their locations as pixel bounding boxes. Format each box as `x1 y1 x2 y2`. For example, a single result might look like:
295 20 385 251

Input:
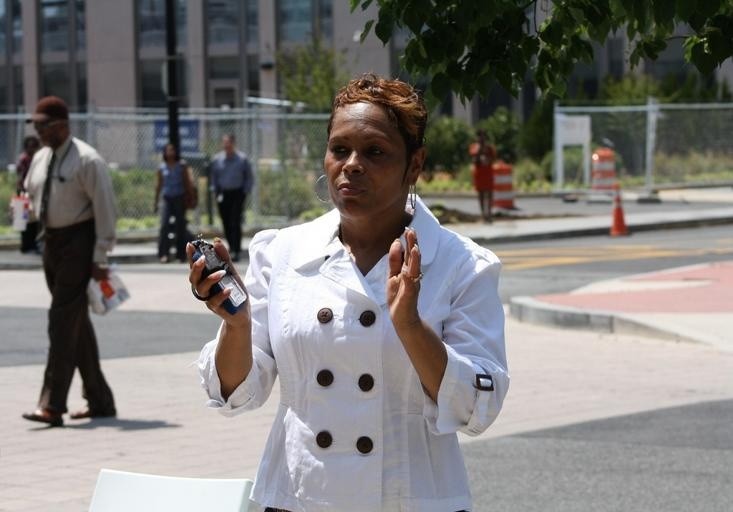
610 182 630 237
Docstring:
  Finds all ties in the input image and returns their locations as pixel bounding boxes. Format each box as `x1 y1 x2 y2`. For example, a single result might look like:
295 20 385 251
39 151 57 227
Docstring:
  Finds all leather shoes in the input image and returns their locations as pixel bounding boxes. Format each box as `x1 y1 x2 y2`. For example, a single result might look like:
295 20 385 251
70 406 117 419
22 407 63 426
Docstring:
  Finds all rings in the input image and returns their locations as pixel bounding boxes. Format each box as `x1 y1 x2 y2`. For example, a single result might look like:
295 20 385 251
411 273 423 283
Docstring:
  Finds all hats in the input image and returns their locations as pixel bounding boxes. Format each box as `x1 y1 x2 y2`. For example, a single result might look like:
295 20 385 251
24 95 70 124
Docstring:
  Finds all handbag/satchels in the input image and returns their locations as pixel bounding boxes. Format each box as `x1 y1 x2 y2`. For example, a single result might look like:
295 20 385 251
185 187 198 209
89 272 131 316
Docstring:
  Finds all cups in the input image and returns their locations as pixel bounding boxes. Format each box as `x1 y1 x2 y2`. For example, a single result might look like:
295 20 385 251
10 197 30 232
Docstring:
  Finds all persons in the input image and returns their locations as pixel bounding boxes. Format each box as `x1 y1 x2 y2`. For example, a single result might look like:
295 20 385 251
16 95 119 426
153 134 198 263
468 128 496 214
185 73 510 512
208 132 253 261
